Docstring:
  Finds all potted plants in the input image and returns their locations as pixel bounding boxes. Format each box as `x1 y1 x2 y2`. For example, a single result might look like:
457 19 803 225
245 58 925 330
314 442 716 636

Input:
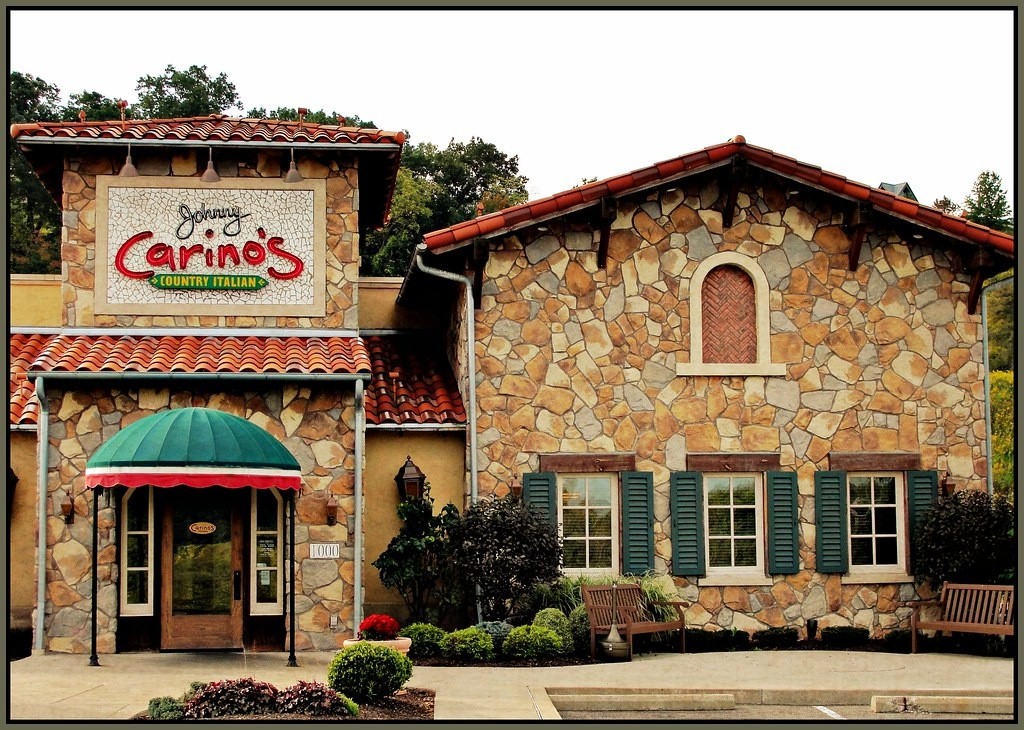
343 613 412 659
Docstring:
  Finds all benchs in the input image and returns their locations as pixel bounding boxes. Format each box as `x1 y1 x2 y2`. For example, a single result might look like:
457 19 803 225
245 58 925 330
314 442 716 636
580 581 689 657
904 578 1017 660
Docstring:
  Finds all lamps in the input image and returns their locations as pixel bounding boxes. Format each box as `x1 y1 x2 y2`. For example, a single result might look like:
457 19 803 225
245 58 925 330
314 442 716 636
200 146 222 184
284 148 304 183
119 143 139 177
